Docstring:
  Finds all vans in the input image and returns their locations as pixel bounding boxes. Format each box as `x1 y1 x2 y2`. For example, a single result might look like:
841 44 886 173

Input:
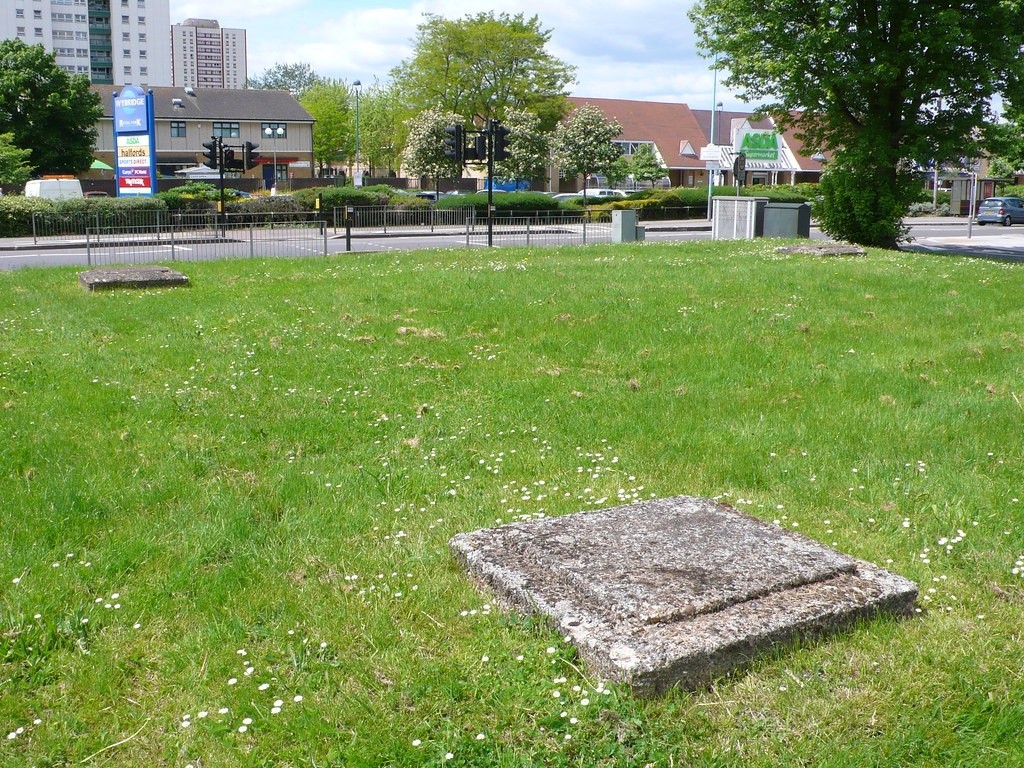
25 179 85 202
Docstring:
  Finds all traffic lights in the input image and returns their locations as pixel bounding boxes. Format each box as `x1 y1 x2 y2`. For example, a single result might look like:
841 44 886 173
444 124 464 162
494 120 512 161
201 135 218 170
225 150 234 169
244 140 261 170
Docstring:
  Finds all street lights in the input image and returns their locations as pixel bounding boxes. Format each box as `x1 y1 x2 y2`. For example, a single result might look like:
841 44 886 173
715 101 723 147
353 79 363 171
265 127 283 187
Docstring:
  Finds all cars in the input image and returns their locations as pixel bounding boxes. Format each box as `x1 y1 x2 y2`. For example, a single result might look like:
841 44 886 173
977 197 1024 226
453 188 624 202
84 191 109 200
